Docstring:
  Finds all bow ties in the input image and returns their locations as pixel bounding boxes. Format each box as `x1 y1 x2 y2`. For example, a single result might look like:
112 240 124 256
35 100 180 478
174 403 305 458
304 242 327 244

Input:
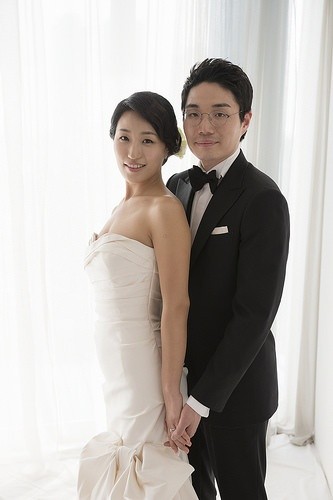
187 164 218 195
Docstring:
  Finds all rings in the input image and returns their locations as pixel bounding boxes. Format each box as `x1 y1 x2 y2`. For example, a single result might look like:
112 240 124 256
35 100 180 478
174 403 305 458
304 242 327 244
170 428 175 432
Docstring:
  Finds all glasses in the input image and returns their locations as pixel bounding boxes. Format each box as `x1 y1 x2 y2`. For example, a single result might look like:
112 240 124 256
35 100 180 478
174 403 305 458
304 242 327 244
183 109 243 127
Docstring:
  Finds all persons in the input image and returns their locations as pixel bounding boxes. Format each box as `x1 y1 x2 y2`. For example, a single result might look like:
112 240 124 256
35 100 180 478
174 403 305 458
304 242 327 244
77 91 199 500
164 58 291 500
